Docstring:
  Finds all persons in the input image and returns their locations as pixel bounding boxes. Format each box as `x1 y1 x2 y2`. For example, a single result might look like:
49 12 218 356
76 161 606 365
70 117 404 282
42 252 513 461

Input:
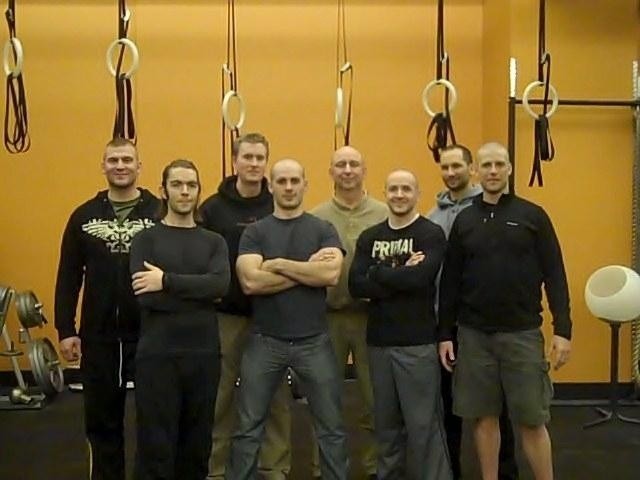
438 141 574 480
308 145 388 479
129 160 232 479
55 138 167 480
348 170 455 477
424 145 520 479
222 158 348 480
195 133 295 480
